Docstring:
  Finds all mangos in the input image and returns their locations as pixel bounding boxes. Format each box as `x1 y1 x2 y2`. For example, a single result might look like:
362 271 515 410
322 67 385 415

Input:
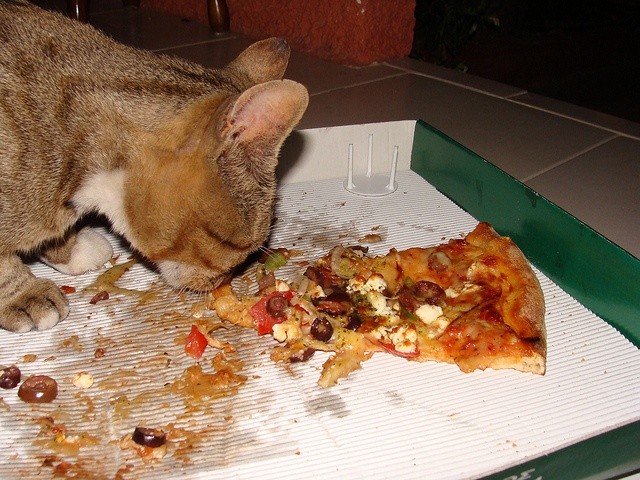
210 219 547 387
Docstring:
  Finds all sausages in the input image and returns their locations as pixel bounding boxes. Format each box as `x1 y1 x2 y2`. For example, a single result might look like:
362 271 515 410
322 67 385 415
0 0 311 334
